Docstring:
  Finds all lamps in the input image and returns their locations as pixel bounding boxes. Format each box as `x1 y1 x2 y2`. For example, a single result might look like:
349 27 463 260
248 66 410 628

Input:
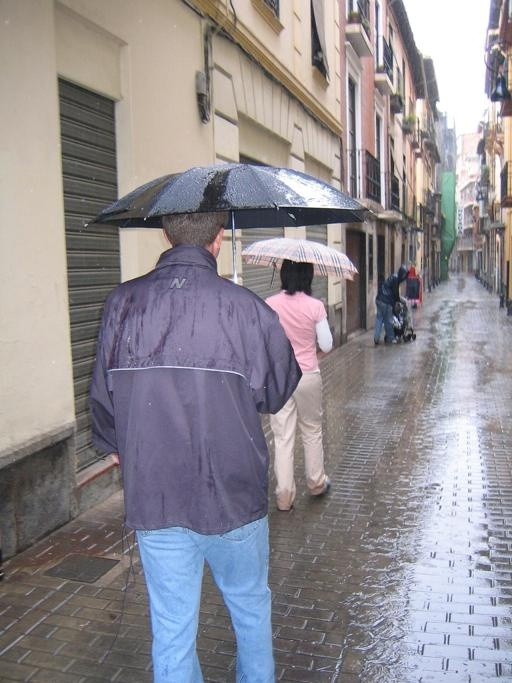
484 38 512 101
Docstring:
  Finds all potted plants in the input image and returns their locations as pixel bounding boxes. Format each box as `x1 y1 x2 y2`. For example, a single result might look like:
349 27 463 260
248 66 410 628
350 11 361 24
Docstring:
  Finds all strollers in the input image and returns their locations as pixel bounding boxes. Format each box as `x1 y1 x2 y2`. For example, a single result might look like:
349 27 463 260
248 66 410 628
383 297 417 343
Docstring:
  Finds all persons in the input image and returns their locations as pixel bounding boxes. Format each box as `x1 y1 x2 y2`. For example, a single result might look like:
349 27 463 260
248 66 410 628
263 257 332 511
373 263 411 345
87 209 304 682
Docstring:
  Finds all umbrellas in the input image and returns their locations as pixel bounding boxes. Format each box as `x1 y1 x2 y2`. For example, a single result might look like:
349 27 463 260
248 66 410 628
88 163 369 284
240 235 360 282
